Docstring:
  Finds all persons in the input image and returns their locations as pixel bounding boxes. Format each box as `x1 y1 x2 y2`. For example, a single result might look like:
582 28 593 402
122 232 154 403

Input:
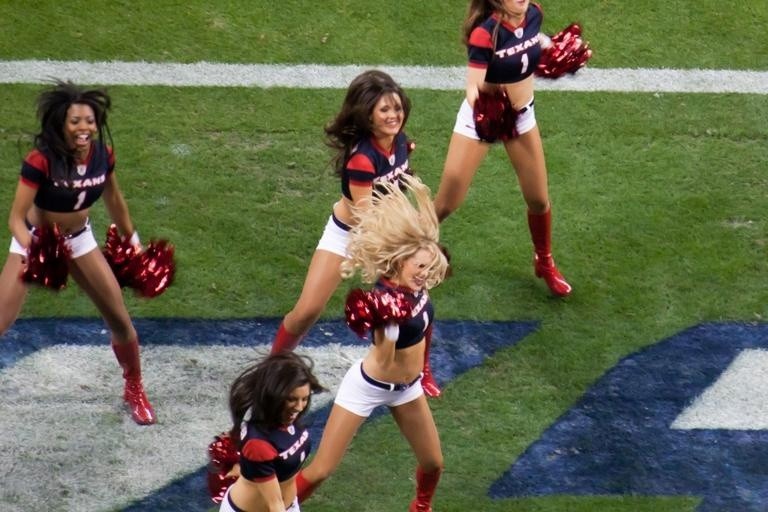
267 69 444 401
434 1 573 302
297 170 448 512
220 349 328 511
1 76 161 428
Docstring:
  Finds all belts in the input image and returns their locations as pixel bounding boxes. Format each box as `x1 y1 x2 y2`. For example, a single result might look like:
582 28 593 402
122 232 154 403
25 218 90 240
519 98 534 113
228 494 245 511
360 363 421 391
331 210 357 234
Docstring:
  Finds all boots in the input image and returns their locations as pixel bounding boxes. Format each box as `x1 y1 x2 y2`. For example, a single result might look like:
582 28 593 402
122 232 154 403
526 202 571 294
270 320 303 356
409 467 444 511
423 323 441 397
112 334 155 425
